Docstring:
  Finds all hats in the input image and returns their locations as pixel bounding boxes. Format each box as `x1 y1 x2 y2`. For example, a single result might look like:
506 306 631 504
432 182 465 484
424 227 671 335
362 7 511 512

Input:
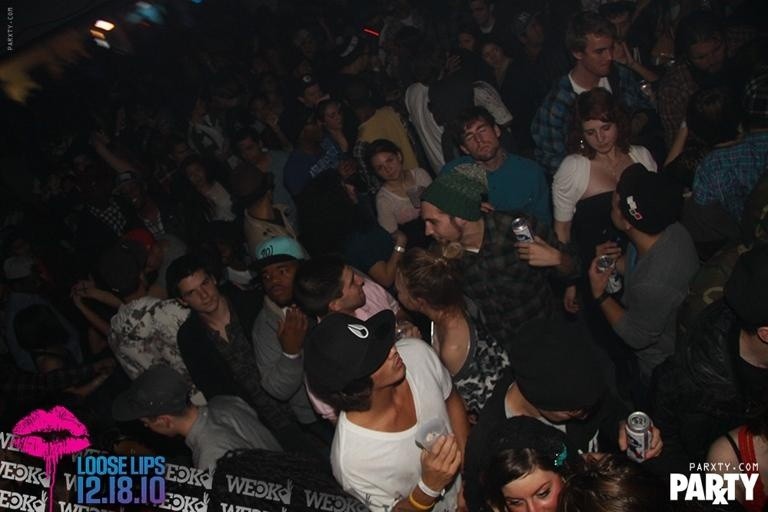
112 170 148 197
110 364 189 421
480 415 569 470
724 245 767 331
515 324 609 412
104 254 139 293
295 73 318 95
510 8 543 35
618 161 683 235
255 236 305 266
305 311 397 388
420 154 488 222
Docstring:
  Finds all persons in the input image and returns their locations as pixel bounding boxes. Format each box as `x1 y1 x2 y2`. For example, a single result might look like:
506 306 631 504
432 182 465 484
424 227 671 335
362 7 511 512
0 0 768 510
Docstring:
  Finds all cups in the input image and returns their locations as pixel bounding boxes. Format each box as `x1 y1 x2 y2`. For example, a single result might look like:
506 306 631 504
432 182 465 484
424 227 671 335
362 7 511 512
414 419 451 452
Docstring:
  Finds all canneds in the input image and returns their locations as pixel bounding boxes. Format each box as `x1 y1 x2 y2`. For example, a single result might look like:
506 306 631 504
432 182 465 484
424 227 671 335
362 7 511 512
595 254 623 295
624 411 654 463
511 216 534 243
639 79 652 97
